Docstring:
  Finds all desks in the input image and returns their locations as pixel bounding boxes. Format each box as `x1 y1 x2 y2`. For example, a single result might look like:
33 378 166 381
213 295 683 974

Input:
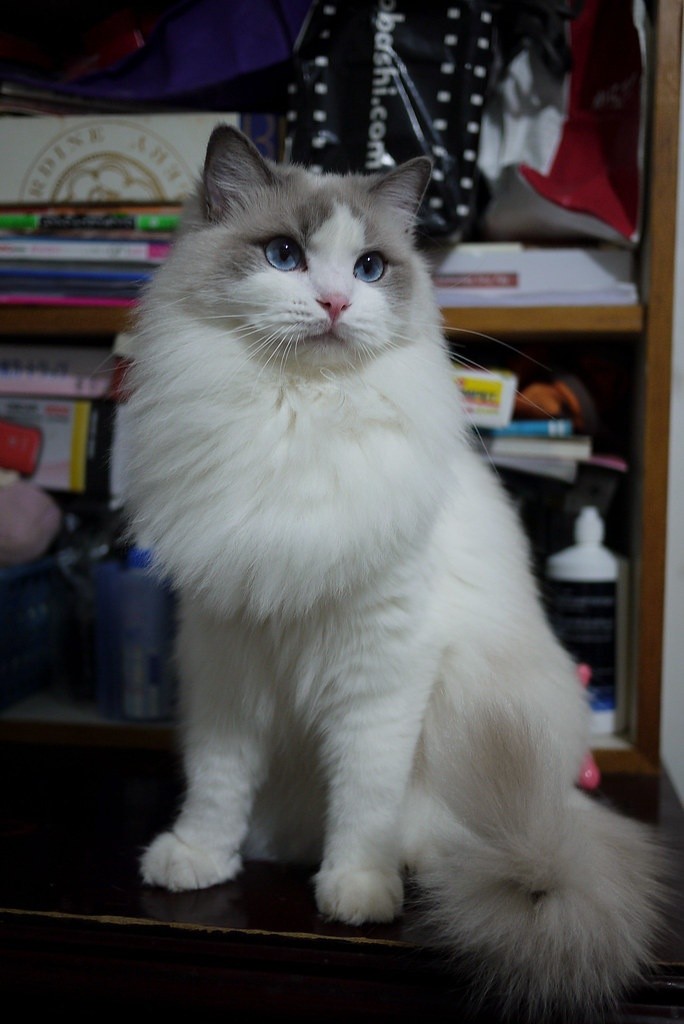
0 740 684 1024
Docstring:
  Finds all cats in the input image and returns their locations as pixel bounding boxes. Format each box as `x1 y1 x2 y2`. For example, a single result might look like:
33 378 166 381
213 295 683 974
109 122 684 1024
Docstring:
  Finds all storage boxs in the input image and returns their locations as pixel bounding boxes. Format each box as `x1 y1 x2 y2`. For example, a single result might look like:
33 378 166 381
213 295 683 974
0 396 116 494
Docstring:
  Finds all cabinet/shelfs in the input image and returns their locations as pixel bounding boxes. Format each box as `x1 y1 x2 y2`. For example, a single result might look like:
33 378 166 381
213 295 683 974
0 0 684 779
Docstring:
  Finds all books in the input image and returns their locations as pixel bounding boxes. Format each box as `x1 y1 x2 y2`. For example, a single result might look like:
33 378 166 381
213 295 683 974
468 418 628 486
424 242 527 289
0 201 184 308
